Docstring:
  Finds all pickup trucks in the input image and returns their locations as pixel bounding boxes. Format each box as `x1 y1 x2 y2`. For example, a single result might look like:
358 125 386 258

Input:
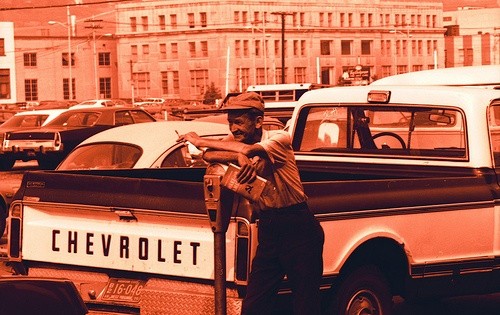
3 83 500 315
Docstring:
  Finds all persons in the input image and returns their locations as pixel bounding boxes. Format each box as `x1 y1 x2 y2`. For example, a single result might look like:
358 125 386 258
175 92 325 315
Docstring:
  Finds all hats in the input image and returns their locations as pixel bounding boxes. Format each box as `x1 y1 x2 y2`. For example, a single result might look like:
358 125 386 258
223 92 265 113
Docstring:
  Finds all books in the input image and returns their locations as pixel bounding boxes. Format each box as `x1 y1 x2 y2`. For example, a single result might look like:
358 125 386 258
218 163 267 203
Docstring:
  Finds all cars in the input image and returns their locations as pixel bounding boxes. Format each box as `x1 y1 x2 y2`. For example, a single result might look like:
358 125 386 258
0 62 500 245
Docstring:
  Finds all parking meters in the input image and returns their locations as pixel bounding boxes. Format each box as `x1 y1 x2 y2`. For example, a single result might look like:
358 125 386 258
203 163 234 315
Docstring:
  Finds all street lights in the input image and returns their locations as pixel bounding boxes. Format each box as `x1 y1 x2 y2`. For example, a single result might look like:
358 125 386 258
390 30 410 73
93 28 113 101
244 25 268 85
47 5 72 100
